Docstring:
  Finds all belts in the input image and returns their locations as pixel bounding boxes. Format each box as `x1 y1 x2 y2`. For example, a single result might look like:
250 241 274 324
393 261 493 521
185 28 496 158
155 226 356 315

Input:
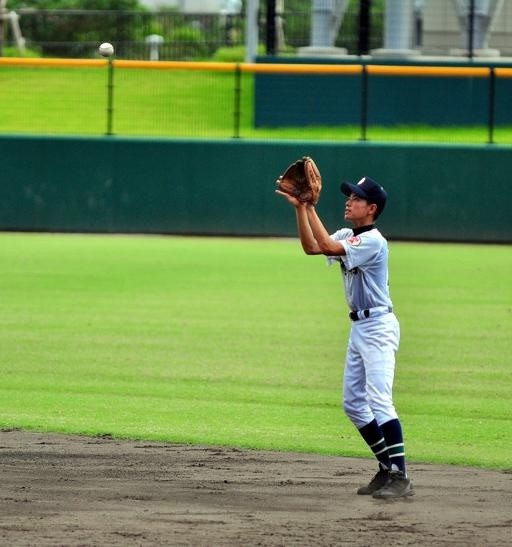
349 310 370 320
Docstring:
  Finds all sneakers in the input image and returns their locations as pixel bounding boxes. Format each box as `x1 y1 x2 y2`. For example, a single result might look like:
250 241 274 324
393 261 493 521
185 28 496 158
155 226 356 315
357 463 388 494
372 471 416 498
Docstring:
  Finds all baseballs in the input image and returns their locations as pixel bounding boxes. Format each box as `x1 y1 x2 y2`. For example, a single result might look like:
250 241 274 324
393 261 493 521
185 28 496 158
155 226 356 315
98 43 115 58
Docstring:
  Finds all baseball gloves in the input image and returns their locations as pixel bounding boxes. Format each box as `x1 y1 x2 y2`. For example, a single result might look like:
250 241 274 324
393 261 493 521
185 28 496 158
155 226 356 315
278 157 322 206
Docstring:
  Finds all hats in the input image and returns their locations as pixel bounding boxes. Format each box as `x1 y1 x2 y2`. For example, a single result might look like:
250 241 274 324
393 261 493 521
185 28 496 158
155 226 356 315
340 177 386 207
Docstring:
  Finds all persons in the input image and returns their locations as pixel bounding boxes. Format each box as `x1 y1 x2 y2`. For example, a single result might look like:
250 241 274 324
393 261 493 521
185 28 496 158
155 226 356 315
270 155 418 499
